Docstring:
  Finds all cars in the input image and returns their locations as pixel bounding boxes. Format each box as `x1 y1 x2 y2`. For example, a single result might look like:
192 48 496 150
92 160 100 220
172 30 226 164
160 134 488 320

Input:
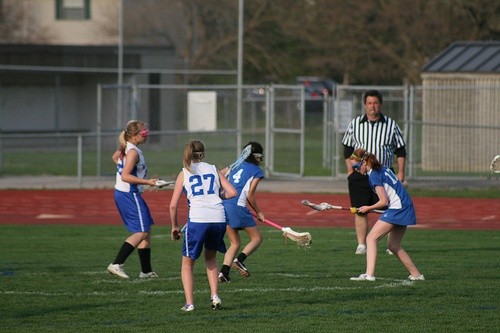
290 76 339 111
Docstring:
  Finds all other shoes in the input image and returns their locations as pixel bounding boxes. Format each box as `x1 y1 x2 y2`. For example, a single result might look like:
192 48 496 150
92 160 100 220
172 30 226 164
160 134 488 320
180 304 195 311
408 274 425 281
355 245 366 254
350 274 376 281
212 297 221 310
106 263 129 278
231 257 250 277
386 248 393 255
139 271 159 278
218 272 231 284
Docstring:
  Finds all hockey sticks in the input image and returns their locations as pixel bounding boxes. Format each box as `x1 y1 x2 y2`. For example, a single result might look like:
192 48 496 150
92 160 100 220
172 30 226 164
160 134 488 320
149 178 176 193
298 199 386 216
176 223 187 239
490 154 500 175
251 211 313 252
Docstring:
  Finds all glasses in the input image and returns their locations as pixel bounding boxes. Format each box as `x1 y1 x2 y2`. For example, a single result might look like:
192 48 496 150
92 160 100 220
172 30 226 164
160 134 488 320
352 164 361 170
142 128 150 137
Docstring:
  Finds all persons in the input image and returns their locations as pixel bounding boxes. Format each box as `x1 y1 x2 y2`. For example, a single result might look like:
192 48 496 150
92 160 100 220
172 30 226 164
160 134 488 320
107 120 162 280
169 139 239 312
217 141 265 282
342 90 407 256
350 148 426 281
